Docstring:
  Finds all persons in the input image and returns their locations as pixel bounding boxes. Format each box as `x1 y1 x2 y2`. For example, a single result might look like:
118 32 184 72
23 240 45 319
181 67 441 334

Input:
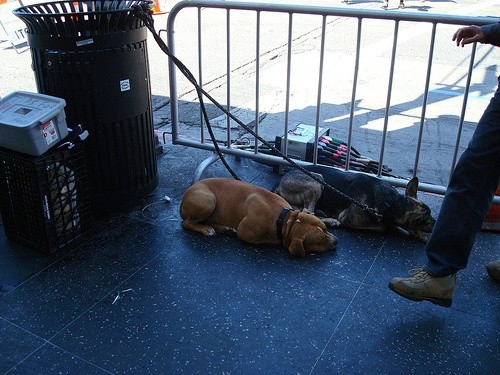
389 22 500 307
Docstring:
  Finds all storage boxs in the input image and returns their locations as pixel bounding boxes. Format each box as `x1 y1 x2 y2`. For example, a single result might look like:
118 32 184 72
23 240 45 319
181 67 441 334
0 92 68 155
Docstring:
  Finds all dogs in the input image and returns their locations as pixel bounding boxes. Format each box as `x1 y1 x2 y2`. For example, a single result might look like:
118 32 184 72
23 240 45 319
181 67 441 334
270 165 436 245
180 178 339 258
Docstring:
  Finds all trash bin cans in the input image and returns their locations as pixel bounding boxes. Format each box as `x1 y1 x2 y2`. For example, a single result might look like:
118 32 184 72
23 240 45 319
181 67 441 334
10 0 158 209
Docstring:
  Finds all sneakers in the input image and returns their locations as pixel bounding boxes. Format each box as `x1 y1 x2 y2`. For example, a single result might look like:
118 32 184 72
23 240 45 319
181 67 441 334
389 269 456 307
486 260 500 282
